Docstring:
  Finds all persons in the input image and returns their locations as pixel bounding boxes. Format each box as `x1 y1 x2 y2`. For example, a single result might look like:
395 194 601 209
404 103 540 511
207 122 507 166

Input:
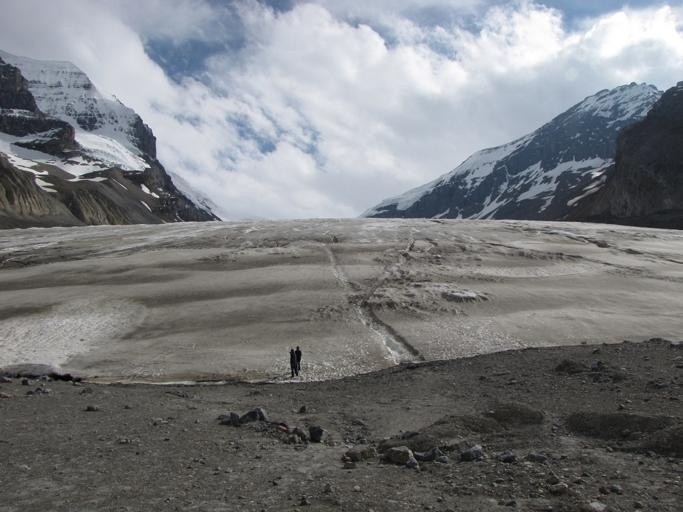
289 348 298 378
295 346 302 370
